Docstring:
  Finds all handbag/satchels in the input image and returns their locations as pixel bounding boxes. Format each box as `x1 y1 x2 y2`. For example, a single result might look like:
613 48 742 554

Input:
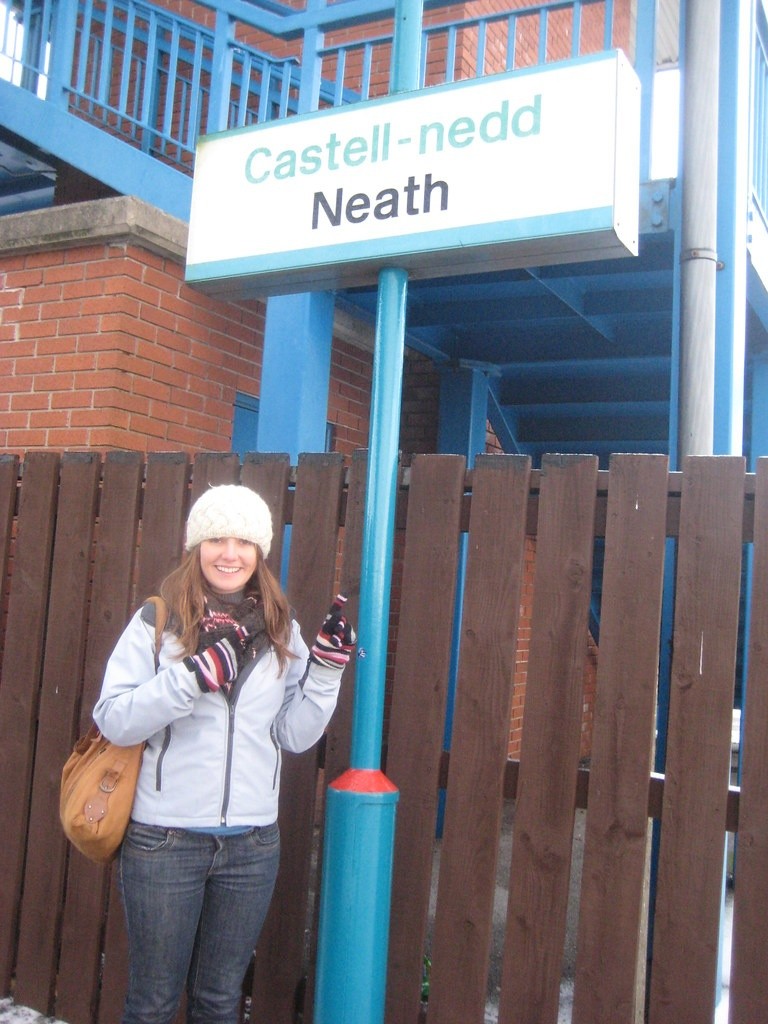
58 596 169 860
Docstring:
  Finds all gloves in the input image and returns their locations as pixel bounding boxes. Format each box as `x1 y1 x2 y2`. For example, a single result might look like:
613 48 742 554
183 623 253 693
309 592 357 669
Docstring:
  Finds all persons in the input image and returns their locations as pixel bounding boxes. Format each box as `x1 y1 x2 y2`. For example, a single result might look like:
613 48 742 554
93 485 357 1024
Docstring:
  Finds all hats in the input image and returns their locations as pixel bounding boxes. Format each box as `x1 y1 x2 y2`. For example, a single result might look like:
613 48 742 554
185 482 273 562
